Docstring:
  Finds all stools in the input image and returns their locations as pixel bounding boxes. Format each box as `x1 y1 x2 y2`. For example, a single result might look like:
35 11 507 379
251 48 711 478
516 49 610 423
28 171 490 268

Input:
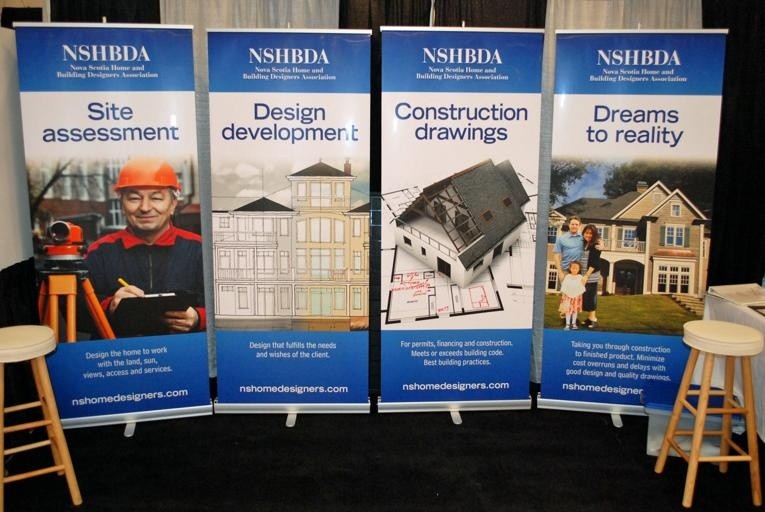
654 319 764 508
0 324 83 512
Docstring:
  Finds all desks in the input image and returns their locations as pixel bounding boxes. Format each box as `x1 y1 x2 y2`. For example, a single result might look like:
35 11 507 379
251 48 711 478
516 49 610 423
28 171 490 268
687 294 765 441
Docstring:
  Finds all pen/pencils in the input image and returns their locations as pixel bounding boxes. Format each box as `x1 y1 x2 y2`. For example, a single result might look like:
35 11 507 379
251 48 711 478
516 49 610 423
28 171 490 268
118 278 128 285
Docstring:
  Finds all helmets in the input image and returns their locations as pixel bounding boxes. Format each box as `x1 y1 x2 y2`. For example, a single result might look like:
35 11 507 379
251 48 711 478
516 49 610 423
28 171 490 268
112 157 183 191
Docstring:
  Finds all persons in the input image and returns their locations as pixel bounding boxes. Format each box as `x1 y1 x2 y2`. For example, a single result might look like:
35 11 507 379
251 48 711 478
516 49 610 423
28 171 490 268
553 216 585 327
580 224 601 329
56 159 207 338
560 262 586 331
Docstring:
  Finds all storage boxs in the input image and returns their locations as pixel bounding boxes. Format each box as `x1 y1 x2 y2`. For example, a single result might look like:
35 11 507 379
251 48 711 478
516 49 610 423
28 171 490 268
640 378 746 466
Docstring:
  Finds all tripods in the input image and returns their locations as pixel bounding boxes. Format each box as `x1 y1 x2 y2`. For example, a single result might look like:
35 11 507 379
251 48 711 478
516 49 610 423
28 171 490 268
37 263 116 344
